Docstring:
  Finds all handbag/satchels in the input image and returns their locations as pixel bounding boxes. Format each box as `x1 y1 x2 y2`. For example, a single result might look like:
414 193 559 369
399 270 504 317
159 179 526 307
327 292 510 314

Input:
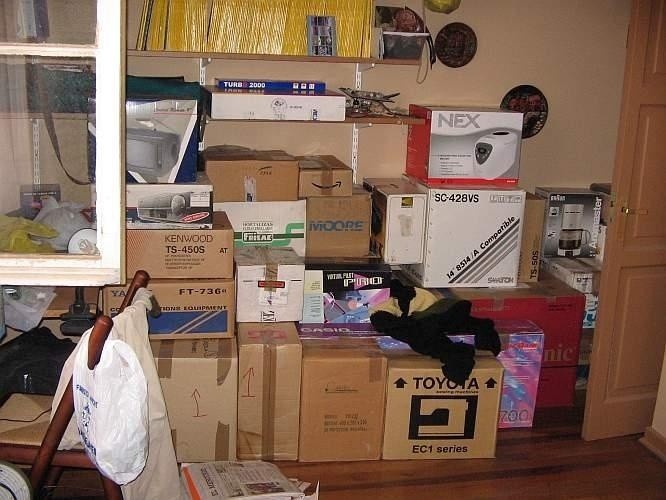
23 63 96 185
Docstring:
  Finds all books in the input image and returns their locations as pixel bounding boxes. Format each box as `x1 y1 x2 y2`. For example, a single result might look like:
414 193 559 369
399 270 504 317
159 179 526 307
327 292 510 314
213 77 327 92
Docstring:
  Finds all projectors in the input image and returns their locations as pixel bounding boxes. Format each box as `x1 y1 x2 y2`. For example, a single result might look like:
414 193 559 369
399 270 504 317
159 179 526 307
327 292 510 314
136 191 213 225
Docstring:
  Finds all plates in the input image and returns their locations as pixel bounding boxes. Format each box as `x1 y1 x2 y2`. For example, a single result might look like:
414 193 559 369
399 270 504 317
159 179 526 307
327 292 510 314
499 84 548 139
433 21 479 68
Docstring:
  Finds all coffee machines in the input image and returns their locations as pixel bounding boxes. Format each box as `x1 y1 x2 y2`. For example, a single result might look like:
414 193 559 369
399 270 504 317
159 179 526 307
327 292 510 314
557 204 591 258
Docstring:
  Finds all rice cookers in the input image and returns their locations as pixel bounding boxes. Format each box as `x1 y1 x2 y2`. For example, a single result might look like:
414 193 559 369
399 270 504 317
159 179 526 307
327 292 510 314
474 131 519 181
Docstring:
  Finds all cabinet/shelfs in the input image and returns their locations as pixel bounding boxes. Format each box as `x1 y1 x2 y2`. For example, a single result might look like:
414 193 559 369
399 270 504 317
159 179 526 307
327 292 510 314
0 40 428 128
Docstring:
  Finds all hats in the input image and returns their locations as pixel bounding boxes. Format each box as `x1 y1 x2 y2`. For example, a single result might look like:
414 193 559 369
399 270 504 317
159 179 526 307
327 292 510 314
0 459 32 500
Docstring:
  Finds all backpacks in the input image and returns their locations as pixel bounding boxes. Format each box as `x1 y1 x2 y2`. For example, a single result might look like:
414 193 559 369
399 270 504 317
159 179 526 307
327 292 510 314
0 326 77 404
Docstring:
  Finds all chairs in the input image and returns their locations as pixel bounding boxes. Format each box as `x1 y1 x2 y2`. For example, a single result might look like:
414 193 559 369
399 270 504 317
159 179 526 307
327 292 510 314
0 268 152 500
